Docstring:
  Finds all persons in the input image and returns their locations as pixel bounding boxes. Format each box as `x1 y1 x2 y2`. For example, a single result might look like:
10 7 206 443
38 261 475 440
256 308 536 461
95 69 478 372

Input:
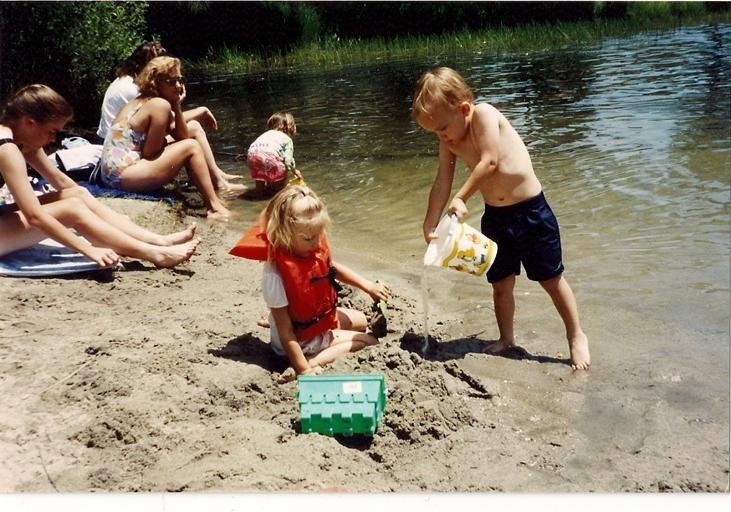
95 39 248 192
0 82 203 271
245 110 305 199
410 64 593 374
99 55 242 221
256 182 393 384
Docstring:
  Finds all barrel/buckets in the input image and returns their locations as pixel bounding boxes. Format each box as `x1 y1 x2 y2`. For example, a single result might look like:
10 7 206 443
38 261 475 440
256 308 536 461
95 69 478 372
423 210 501 280
